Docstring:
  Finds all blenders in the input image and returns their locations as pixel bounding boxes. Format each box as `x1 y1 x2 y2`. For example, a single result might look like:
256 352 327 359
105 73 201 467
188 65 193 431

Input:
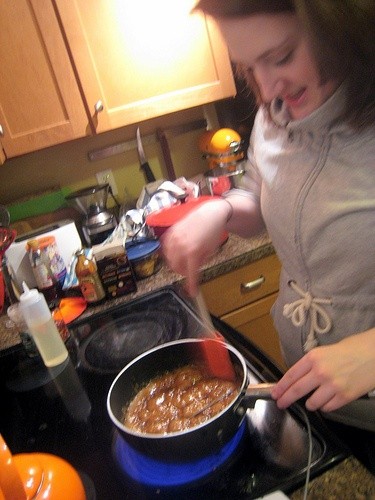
66 183 122 247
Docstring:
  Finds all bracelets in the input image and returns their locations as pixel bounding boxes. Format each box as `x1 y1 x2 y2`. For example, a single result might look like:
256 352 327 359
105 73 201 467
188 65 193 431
223 198 233 224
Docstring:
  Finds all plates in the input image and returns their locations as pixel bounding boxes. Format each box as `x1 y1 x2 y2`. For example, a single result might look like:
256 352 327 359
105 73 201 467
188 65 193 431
50 298 87 322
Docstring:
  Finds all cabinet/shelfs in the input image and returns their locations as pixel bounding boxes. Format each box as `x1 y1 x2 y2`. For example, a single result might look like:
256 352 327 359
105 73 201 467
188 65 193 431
201 252 281 369
0 0 237 159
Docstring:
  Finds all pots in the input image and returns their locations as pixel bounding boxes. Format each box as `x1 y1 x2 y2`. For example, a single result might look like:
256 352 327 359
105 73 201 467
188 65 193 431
205 165 244 196
108 335 278 465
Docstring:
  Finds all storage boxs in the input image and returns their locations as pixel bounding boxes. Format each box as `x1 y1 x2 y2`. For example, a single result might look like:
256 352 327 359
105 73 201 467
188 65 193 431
94 244 138 302
0 182 76 223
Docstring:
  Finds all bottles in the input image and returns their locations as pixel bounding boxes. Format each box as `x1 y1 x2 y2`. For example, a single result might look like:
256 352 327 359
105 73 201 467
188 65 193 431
19 279 67 367
8 303 43 360
26 236 66 311
74 250 105 307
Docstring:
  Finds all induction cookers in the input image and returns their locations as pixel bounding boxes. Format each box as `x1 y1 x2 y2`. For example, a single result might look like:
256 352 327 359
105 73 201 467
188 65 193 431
0 284 352 500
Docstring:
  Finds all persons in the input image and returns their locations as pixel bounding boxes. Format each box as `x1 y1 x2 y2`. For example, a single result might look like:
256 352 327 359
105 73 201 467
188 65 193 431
159 0 375 477
